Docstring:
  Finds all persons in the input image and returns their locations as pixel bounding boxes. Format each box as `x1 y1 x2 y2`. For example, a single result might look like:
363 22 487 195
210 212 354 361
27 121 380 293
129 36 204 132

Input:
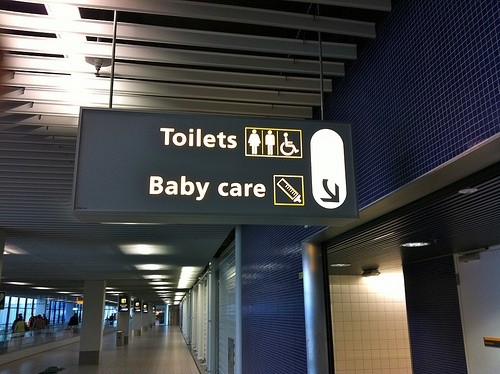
11 309 117 334
